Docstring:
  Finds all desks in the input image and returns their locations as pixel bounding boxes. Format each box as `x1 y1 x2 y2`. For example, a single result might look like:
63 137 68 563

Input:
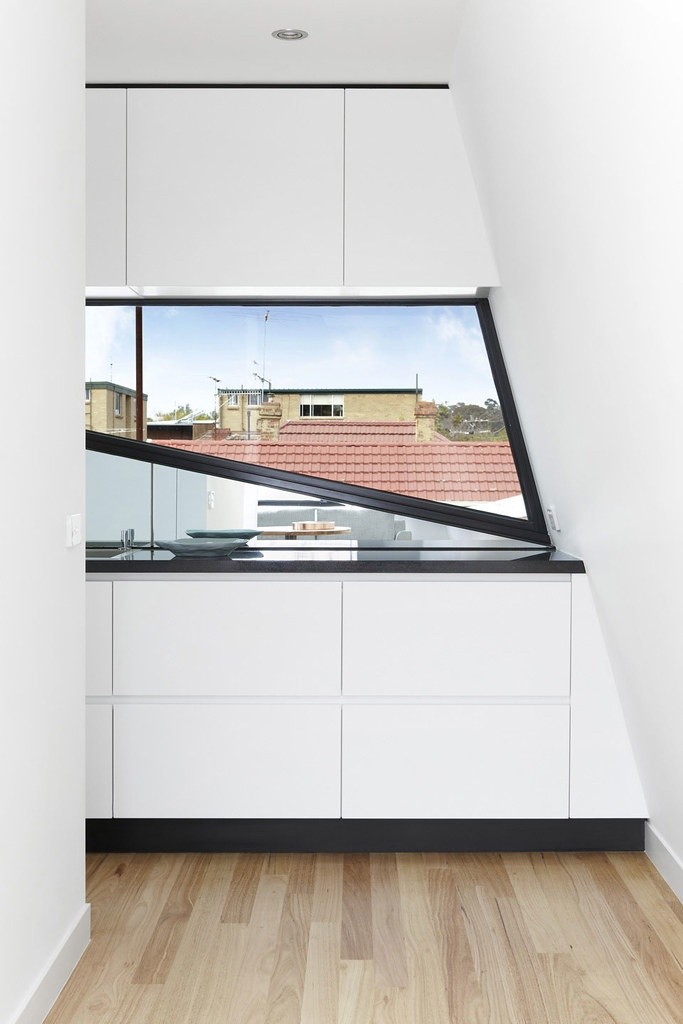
255 525 351 540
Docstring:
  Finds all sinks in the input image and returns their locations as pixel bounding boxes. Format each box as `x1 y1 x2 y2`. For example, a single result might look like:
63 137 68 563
86 549 142 560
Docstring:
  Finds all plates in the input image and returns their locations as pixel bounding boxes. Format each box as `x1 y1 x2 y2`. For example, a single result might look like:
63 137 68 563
186 527 264 542
165 537 248 557
155 541 169 548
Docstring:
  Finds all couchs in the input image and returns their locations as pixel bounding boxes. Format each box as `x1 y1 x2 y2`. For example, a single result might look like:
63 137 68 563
258 506 412 540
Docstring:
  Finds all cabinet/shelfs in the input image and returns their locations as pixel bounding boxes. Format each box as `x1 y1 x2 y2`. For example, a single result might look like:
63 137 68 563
85 577 569 819
